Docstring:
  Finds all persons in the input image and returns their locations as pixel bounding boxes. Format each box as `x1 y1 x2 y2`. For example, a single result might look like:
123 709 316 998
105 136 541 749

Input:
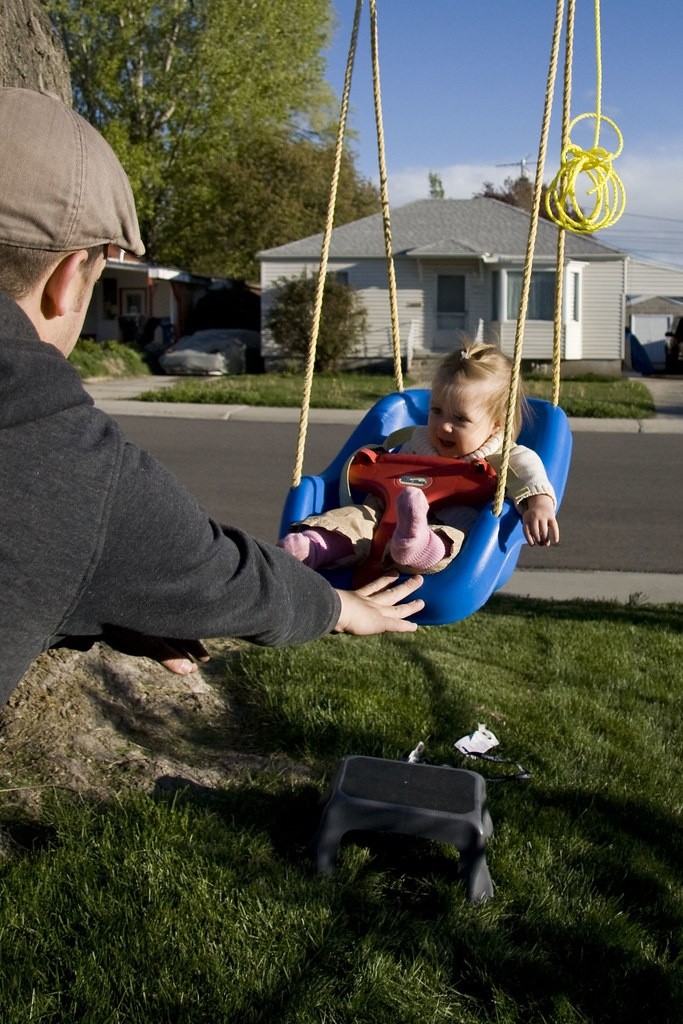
276 342 560 574
0 83 426 727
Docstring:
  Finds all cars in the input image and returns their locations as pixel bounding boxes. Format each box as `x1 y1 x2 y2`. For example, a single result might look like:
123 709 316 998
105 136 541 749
666 317 683 367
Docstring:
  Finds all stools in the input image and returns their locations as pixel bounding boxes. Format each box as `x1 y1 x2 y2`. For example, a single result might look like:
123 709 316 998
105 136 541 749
317 755 494 898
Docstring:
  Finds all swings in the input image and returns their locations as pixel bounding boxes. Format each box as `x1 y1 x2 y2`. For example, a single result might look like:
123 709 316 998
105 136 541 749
274 0 578 626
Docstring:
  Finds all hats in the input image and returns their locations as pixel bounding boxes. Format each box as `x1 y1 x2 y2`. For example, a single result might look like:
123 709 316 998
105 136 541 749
0 87 145 257
